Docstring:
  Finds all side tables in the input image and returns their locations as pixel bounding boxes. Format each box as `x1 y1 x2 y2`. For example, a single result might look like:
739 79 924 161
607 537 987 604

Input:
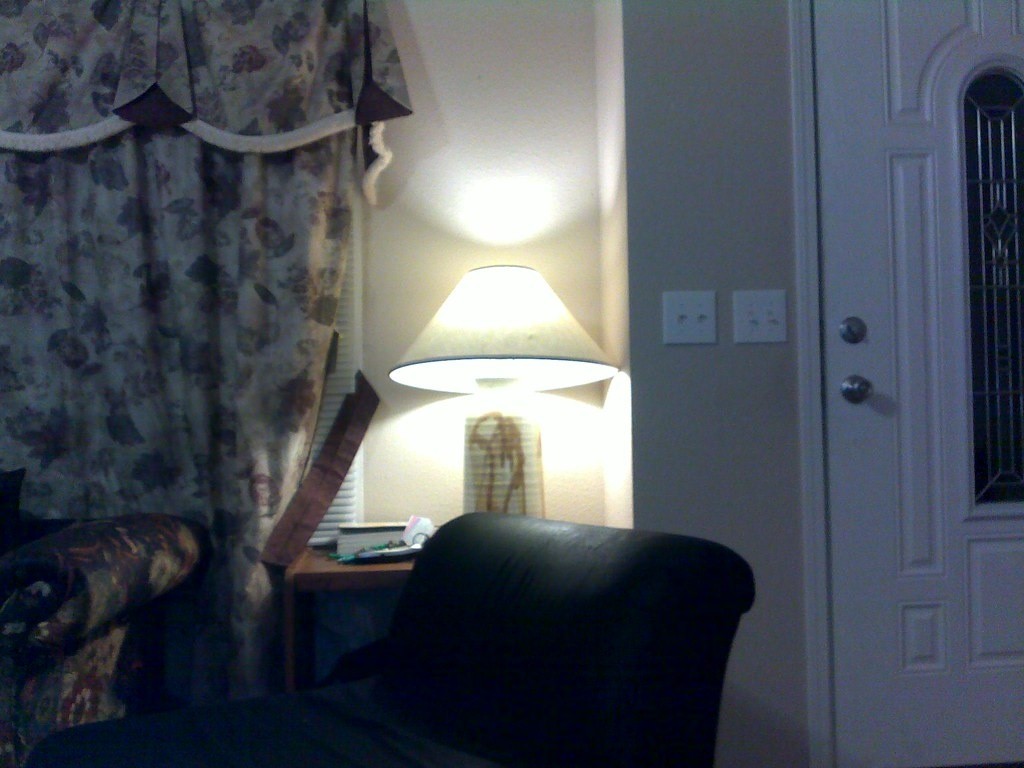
288 547 417 703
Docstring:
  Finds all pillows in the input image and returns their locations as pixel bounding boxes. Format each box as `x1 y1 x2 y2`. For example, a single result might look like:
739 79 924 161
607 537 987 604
0 468 26 557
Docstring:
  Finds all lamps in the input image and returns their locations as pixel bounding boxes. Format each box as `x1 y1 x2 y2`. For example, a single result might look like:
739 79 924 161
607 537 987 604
388 264 622 519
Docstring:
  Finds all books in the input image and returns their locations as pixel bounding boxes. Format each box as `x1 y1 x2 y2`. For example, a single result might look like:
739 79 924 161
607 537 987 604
337 522 406 555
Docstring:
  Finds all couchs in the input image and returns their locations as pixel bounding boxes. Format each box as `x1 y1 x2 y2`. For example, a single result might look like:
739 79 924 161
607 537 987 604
0 517 206 768
22 512 758 768
